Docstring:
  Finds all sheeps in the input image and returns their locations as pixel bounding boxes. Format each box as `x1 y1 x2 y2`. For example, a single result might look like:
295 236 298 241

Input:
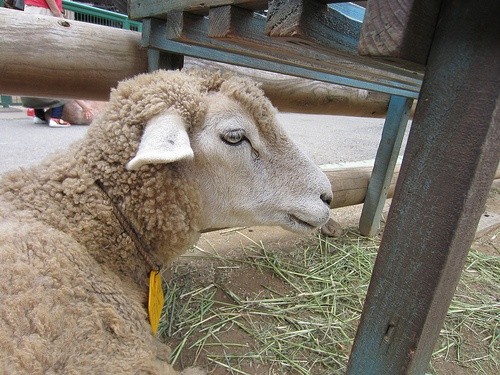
0 66 333 374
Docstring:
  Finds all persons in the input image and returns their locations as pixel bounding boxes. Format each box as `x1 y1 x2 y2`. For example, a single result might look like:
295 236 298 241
23 0 97 127
74 99 96 119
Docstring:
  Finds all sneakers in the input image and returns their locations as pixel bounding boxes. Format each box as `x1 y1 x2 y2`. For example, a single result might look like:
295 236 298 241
34 116 47 124
49 118 71 127
